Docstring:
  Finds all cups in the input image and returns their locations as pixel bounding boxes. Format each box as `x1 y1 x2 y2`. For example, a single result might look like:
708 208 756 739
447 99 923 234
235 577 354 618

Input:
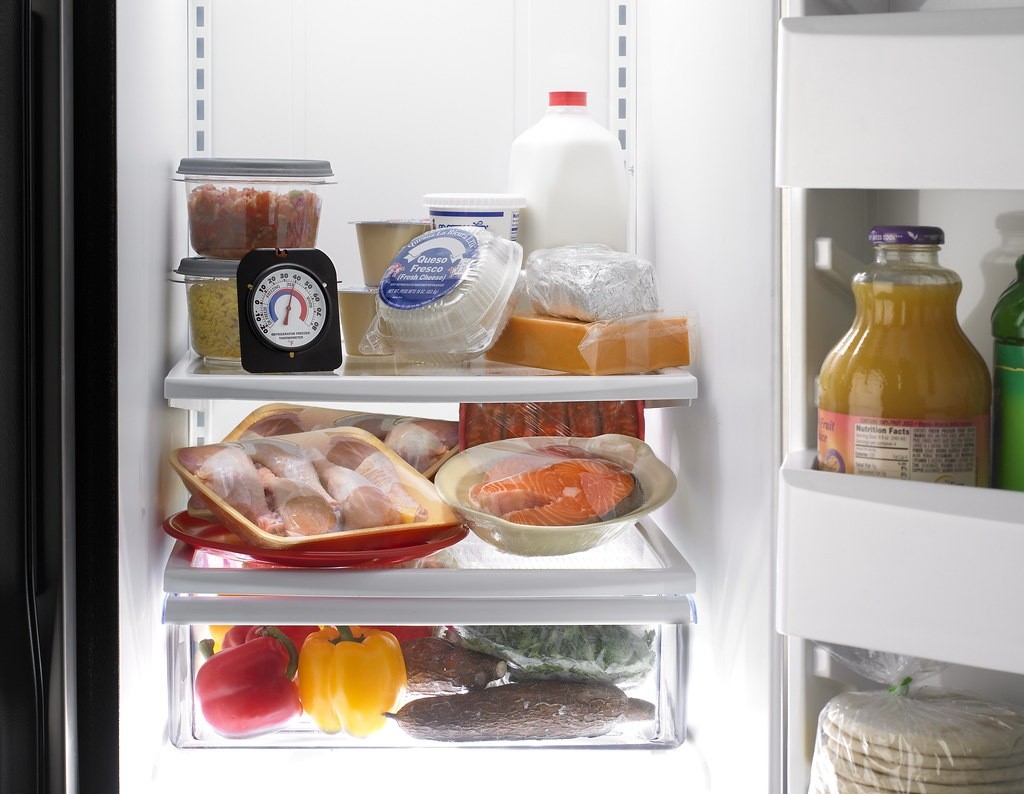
337 218 433 358
422 191 526 243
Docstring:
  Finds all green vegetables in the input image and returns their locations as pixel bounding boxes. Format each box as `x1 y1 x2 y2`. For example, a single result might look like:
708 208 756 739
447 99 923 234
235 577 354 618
446 624 656 687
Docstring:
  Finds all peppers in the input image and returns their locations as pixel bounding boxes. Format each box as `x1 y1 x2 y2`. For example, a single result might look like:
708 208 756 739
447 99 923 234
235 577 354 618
196 624 437 739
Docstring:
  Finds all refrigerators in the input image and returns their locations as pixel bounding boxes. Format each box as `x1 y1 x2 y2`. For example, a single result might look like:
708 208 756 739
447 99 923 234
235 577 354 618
110 0 1024 792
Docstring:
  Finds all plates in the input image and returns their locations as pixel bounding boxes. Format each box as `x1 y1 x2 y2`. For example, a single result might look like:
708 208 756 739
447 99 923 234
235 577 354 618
162 402 471 571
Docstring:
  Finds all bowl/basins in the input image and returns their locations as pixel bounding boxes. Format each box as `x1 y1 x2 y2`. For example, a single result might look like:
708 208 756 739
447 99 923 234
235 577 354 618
434 432 678 557
176 157 339 259
172 257 244 370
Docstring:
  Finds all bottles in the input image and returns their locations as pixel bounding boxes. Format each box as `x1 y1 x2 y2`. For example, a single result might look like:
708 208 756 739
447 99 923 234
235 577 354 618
509 90 630 268
990 253 1024 496
816 225 994 489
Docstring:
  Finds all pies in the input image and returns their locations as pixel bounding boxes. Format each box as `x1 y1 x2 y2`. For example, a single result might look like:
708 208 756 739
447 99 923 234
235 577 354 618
810 693 1024 794
